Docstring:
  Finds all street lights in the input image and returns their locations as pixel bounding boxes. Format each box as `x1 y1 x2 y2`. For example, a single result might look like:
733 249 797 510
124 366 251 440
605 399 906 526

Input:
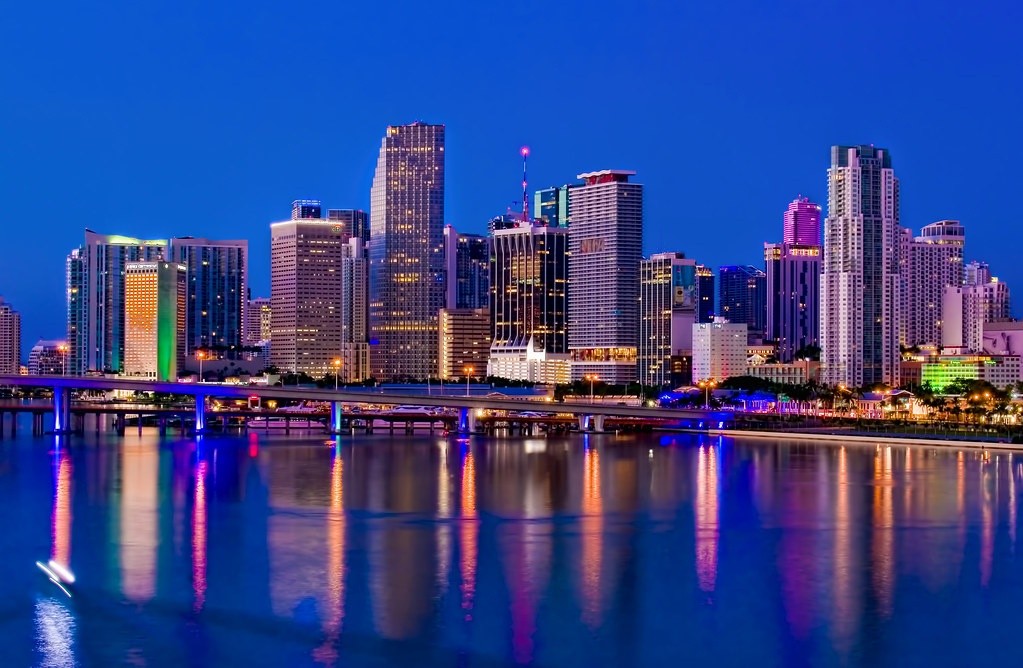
699 381 714 411
464 367 472 395
59 345 67 376
197 352 204 382
335 359 340 391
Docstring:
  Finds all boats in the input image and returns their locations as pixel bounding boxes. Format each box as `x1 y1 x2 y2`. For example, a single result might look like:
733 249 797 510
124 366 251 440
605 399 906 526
353 404 445 429
246 399 326 430
